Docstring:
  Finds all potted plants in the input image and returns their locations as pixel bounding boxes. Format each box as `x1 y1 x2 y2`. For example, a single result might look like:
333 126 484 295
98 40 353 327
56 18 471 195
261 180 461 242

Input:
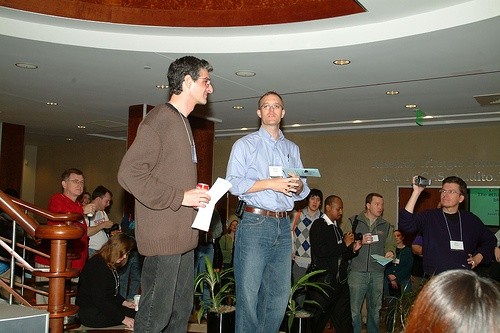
283 270 336 333
193 255 236 333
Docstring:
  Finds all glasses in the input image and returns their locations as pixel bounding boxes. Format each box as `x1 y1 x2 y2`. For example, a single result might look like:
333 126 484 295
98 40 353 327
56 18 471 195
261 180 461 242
438 189 460 194
198 77 211 85
69 179 85 185
83 196 90 200
259 103 284 110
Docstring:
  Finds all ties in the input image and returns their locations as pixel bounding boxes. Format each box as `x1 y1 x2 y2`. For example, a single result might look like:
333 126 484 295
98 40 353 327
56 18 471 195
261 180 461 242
334 224 341 241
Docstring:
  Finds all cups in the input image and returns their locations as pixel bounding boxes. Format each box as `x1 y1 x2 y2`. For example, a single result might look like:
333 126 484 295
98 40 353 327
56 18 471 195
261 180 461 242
87 213 93 217
133 295 141 311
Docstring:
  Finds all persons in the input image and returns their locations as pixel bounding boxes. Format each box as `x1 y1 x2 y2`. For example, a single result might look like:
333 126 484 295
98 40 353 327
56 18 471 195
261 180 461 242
224 92 310 333
194 207 223 303
493 229 500 262
219 220 238 286
309 195 362 333
385 230 413 302
118 56 213 333
292 188 323 307
74 233 136 328
405 269 500 333
399 176 497 279
118 220 145 298
85 186 114 256
34 168 96 328
342 193 397 333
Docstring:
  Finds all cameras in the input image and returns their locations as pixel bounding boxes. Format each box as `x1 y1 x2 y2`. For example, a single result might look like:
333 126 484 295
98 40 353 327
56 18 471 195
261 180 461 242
351 215 363 241
414 176 432 188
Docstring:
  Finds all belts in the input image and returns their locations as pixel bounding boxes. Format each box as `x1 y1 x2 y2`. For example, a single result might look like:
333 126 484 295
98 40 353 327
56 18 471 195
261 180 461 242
244 206 290 217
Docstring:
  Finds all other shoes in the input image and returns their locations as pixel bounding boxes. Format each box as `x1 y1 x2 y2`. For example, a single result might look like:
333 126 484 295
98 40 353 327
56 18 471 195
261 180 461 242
189 314 195 323
203 313 207 320
